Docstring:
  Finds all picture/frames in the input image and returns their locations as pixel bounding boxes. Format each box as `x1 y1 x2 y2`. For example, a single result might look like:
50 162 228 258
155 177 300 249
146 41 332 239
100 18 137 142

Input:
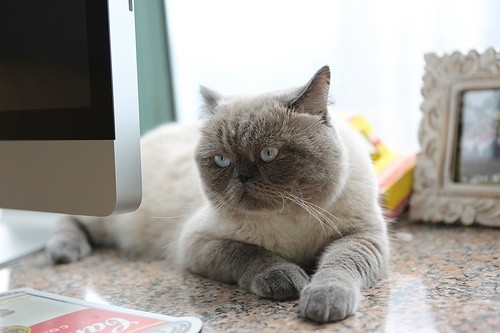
405 46 500 227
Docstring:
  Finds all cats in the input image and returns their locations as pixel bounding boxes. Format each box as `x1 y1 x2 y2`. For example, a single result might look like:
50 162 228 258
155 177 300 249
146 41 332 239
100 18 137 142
44 65 406 324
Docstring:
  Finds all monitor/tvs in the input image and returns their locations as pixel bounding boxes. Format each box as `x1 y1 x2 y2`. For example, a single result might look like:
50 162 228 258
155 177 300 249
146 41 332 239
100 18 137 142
0 0 142 217
